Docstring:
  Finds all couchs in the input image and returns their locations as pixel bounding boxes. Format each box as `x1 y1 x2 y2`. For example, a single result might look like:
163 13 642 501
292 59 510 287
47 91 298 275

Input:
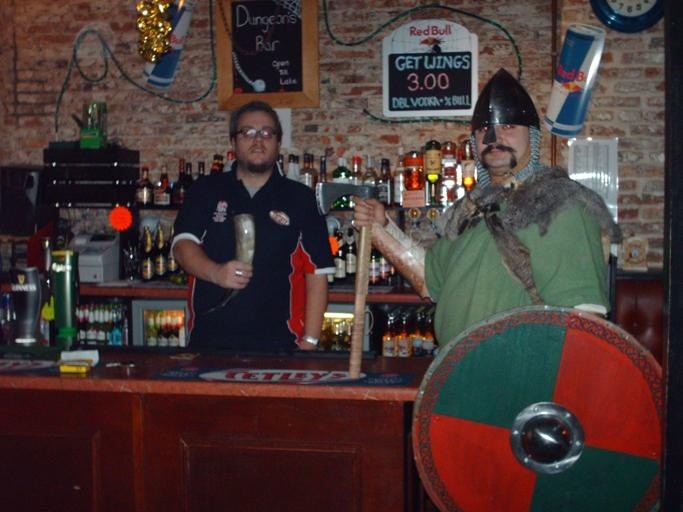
614 279 667 373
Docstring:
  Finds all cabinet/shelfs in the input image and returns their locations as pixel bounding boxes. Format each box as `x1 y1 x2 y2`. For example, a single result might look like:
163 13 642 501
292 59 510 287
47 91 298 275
0 387 420 511
0 216 192 302
326 208 435 307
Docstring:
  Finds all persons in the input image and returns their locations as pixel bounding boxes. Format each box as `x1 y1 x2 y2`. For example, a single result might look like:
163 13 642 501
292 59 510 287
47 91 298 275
169 99 338 355
354 68 613 350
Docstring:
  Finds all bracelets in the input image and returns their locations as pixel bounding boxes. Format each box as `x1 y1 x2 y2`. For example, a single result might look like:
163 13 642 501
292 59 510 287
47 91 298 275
303 336 320 346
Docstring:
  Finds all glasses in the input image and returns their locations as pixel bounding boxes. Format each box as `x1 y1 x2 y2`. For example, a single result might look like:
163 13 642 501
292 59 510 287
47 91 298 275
231 125 283 139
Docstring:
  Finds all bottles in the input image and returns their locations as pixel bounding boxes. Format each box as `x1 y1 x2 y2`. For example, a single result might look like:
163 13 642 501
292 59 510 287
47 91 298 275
319 317 353 351
134 151 235 208
141 220 180 282
381 305 437 357
144 310 185 348
328 226 403 291
77 301 129 347
277 139 476 209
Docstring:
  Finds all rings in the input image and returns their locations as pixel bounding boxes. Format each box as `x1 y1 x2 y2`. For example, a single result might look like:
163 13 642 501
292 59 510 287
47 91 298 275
235 269 242 277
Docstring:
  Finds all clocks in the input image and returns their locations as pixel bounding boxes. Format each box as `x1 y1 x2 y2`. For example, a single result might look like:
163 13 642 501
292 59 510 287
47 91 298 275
590 0 665 33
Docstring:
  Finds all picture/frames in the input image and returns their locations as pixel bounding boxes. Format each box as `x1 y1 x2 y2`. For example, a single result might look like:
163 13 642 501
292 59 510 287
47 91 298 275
212 0 321 111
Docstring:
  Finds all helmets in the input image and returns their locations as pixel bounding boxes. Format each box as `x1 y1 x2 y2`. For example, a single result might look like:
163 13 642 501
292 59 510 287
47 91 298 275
470 67 542 145
